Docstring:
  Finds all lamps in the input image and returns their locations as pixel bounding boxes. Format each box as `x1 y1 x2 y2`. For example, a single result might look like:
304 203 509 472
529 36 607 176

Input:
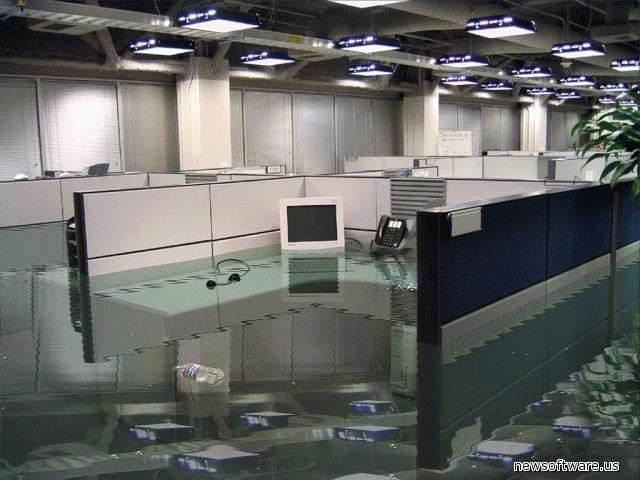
133 398 607 473
129 8 640 106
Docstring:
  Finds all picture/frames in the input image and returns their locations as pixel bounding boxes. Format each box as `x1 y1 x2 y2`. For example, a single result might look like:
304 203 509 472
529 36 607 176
90 242 416 361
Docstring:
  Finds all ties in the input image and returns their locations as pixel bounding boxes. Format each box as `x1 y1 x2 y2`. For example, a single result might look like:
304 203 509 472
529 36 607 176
278 198 345 251
280 254 347 309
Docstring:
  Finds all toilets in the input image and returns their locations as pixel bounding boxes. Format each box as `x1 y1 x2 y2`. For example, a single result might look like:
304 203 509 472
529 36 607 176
376 215 407 247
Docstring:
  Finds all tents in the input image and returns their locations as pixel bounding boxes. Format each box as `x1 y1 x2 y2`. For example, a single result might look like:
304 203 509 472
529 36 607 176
205 258 250 290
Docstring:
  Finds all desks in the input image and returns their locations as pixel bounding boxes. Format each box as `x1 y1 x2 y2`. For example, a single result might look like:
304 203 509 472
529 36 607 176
90 242 416 361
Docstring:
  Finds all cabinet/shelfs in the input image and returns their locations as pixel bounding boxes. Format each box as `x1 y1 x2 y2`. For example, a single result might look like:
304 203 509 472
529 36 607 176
171 363 224 385
